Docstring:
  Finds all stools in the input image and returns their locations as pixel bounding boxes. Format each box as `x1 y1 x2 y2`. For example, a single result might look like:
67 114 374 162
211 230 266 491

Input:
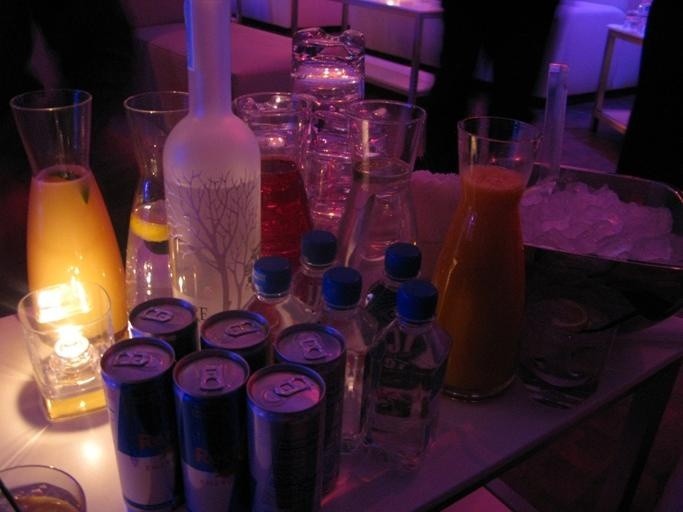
121 22 293 129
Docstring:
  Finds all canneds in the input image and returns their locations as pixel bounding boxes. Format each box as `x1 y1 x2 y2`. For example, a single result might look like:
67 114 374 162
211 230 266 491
100 298 347 511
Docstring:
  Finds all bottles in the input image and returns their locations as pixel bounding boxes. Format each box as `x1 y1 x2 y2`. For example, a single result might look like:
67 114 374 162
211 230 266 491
429 112 547 404
335 96 429 293
305 264 366 458
362 240 425 351
286 21 366 241
161 0 263 327
289 227 340 312
241 254 320 342
8 87 129 343
120 89 191 310
232 88 317 266
367 277 456 471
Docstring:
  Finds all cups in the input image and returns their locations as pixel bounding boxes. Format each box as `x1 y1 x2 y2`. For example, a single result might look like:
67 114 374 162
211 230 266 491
15 281 116 424
511 273 636 412
0 464 89 512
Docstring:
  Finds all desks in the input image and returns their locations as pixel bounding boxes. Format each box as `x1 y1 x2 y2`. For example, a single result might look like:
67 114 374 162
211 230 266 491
0 243 682 512
